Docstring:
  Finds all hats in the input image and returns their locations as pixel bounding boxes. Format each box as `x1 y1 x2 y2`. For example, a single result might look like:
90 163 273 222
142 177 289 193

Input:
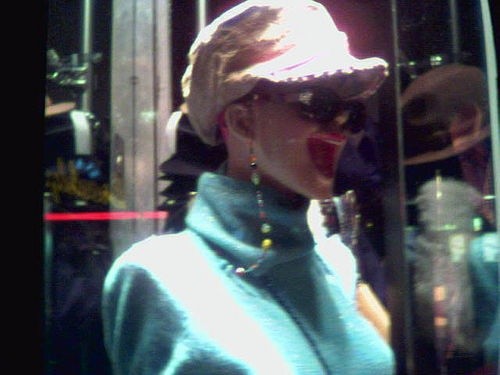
395 64 494 165
179 1 386 143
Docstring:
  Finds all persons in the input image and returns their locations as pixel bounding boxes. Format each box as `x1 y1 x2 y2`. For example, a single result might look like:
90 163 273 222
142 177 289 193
416 153 493 363
100 1 397 375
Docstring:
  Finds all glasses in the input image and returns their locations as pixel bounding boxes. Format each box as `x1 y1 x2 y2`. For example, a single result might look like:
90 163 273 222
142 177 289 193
286 87 366 134
